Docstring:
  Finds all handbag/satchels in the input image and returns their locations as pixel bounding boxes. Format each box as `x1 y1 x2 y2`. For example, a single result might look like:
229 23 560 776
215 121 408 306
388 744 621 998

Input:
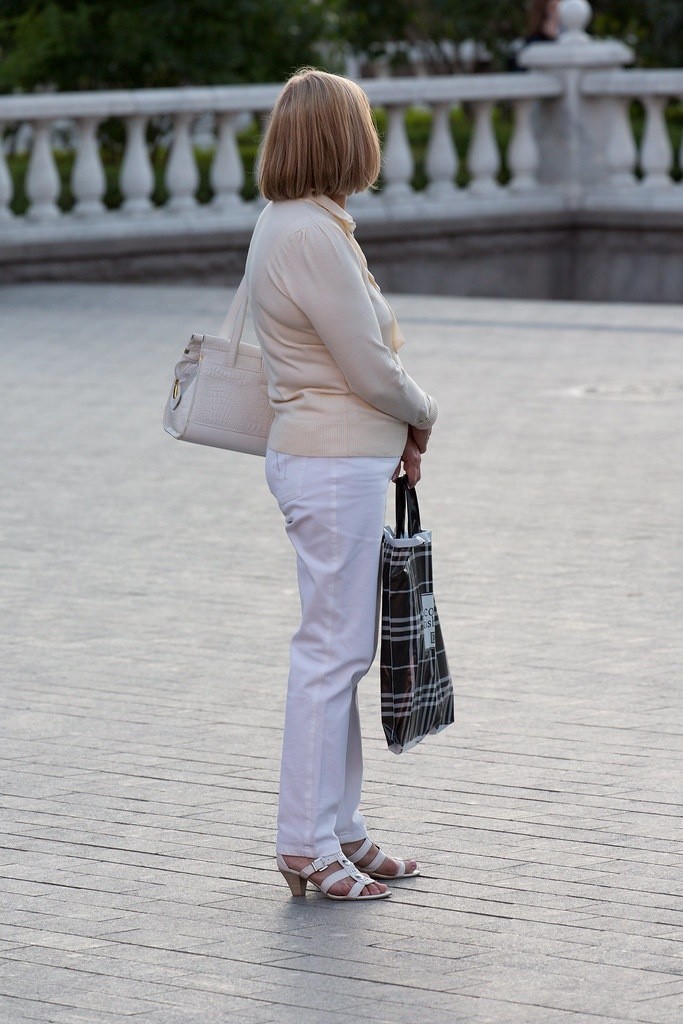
164 272 271 455
381 474 454 755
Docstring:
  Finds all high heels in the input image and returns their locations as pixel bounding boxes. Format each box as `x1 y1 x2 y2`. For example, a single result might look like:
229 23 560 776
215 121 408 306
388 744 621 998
277 850 392 900
348 838 420 879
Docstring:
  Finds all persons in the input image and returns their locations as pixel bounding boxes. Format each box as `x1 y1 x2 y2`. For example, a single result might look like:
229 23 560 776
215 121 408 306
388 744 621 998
164 69 456 904
